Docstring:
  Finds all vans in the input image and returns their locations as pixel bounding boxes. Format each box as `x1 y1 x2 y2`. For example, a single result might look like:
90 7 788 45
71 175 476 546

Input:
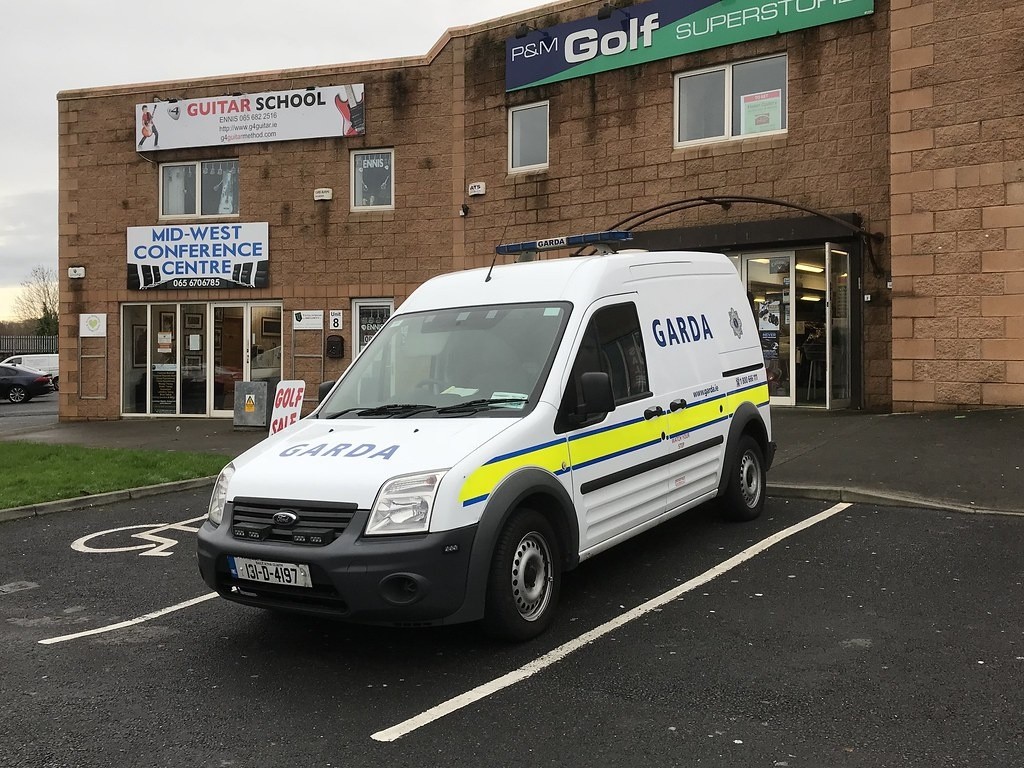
194 227 781 643
0 353 59 391
251 345 281 382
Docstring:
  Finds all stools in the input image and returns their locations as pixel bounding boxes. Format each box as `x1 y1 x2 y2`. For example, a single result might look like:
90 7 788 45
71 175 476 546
802 344 845 401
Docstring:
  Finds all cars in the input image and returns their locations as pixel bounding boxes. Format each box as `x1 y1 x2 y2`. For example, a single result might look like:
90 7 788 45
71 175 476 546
0 361 56 405
182 363 242 393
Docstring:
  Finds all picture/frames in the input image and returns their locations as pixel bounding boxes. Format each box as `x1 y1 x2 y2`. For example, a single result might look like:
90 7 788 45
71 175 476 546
184 313 203 330
131 324 147 368
184 335 203 351
214 307 224 323
184 355 202 371
261 317 281 337
215 326 222 349
159 312 176 340
